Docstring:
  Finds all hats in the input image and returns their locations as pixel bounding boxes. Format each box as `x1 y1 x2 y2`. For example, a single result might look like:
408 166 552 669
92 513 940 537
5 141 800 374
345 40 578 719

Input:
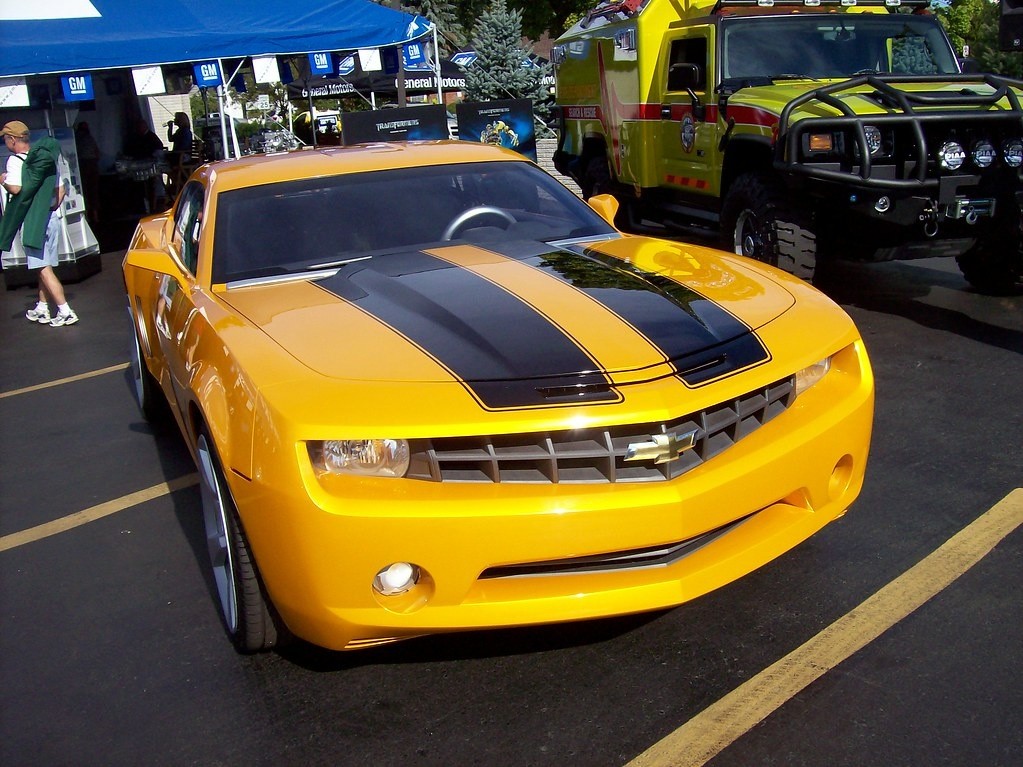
0 121 29 137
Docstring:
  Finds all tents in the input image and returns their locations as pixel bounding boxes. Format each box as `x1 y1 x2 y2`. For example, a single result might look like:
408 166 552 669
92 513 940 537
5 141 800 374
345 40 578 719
287 55 469 132
446 35 550 71
1 1 445 162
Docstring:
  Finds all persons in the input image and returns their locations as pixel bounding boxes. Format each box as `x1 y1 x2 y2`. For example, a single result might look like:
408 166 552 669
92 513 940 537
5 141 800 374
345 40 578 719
0 121 78 327
137 120 165 192
168 112 192 165
76 122 101 222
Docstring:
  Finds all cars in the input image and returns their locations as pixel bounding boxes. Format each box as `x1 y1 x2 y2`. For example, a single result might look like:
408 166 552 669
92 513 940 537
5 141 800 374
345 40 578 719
121 139 875 653
197 112 231 122
381 103 460 139
294 109 341 133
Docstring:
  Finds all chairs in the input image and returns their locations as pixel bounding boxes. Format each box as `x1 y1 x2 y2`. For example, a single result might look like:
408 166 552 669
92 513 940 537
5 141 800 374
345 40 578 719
165 139 204 191
226 171 463 267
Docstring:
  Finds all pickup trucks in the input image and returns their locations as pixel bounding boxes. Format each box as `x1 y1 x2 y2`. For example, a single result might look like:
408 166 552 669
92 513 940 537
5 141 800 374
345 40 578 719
551 0 1023 298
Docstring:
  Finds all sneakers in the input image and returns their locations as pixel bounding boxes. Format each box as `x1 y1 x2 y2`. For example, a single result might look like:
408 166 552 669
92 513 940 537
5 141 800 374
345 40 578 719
49 311 79 327
26 308 50 323
